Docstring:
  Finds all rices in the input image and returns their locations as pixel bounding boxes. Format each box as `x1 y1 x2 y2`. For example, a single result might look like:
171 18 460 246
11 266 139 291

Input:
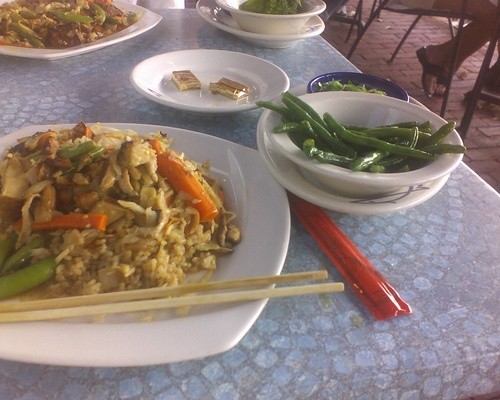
31 129 216 297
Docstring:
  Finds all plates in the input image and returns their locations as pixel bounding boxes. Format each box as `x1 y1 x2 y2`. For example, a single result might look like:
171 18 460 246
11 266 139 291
129 49 290 114
0 0 163 61
196 0 325 48
256 85 452 213
1 124 292 367
307 71 409 103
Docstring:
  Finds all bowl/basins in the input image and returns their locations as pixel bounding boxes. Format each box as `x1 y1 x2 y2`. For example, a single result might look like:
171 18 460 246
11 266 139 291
265 90 464 196
215 0 327 36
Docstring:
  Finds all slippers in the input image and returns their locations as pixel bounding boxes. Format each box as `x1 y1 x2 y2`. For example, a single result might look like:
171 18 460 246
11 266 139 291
416 47 450 99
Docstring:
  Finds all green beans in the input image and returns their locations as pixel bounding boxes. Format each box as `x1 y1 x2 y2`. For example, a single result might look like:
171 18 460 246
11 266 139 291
0 141 105 298
255 91 466 173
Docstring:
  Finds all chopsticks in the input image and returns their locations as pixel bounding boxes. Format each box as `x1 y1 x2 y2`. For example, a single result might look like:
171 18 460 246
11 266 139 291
0 269 345 323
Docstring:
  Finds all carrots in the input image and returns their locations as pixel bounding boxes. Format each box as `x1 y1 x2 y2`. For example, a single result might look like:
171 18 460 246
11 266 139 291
31 215 107 230
152 141 217 224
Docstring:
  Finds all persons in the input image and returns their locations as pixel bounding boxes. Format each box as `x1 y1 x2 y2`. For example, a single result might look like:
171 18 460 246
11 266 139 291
399 0 500 98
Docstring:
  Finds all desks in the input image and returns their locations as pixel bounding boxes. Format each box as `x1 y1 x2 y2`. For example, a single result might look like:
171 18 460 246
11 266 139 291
0 9 500 400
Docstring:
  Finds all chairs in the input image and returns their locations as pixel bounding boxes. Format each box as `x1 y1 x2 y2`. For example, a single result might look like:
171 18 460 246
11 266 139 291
330 0 499 140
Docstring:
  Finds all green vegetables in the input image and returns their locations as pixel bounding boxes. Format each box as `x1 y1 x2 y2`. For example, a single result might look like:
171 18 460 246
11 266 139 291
9 3 106 48
239 0 303 14
318 80 386 94
